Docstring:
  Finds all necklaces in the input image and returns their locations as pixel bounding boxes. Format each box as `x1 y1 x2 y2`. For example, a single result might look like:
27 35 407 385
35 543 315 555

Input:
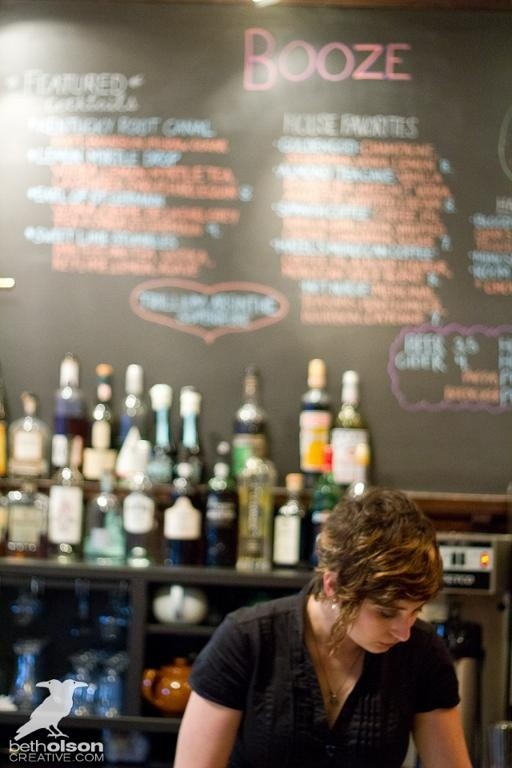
310 623 362 706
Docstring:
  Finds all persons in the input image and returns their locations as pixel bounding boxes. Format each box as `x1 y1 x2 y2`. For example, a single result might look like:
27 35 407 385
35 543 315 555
173 487 472 768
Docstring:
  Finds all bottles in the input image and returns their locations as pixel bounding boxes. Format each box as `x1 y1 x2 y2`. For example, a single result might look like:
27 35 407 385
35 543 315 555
9 572 129 717
1 354 378 579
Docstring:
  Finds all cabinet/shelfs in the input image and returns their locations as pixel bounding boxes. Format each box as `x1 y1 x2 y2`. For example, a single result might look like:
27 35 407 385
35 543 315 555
1 557 309 767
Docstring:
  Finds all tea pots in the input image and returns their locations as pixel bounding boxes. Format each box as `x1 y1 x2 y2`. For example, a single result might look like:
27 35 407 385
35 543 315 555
140 655 198 714
154 584 209 628
